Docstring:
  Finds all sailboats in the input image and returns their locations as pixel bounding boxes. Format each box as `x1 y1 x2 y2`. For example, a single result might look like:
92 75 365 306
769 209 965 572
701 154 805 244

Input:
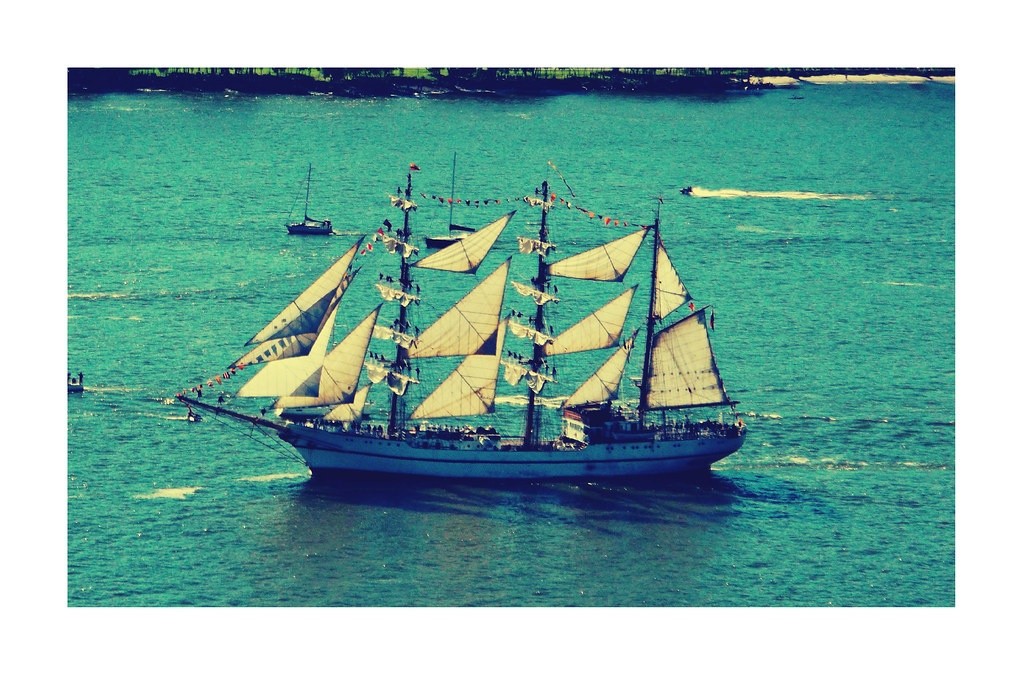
283 162 334 234
425 151 477 247
177 161 745 480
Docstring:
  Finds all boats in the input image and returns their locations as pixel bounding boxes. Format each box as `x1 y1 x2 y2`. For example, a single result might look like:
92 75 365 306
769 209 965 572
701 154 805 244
679 186 694 194
67 370 83 392
